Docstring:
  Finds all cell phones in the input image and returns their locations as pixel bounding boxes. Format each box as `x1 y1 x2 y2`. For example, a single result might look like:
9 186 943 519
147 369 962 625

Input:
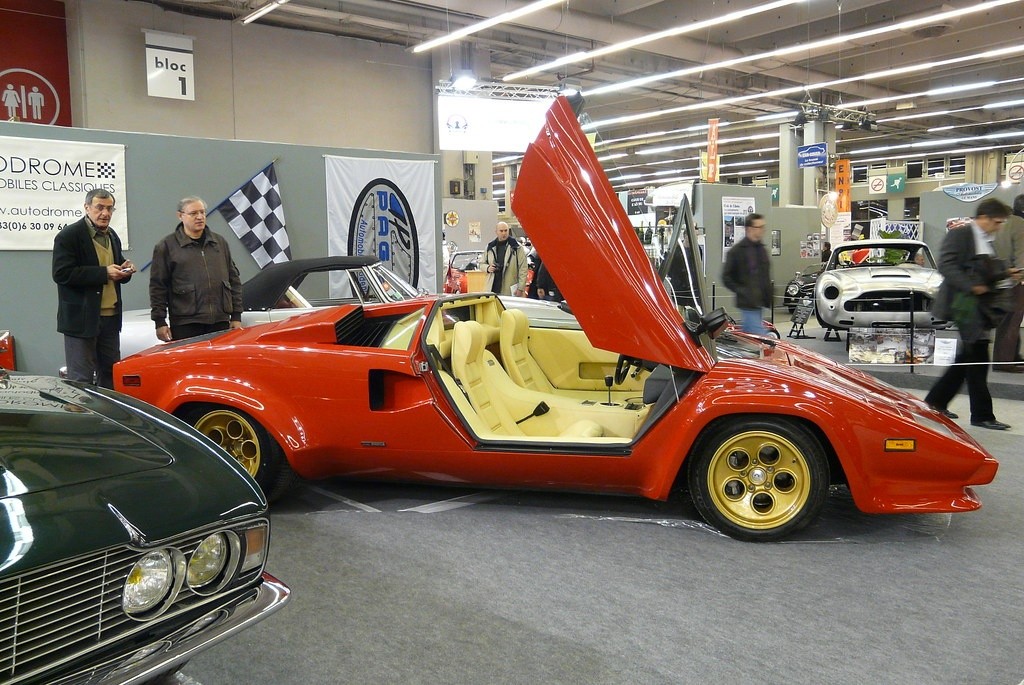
121 268 132 273
1011 268 1024 274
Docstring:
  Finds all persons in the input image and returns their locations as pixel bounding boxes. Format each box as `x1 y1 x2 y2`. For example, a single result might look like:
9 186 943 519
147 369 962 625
51 189 136 390
722 214 775 335
148 197 242 343
913 194 1024 373
442 221 565 302
922 197 1021 430
661 227 706 307
820 243 831 262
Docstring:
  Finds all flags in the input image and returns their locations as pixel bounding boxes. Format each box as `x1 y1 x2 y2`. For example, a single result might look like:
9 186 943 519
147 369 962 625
217 166 293 269
322 156 437 297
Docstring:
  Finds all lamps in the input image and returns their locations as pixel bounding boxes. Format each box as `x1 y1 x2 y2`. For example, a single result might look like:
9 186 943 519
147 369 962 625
241 0 291 24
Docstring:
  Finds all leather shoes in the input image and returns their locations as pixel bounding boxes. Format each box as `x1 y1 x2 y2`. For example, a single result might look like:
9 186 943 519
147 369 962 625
970 418 1011 429
940 408 958 419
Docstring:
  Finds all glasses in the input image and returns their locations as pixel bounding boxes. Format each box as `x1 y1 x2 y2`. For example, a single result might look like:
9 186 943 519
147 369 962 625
989 217 1004 225
748 225 765 229
179 209 207 217
88 202 116 212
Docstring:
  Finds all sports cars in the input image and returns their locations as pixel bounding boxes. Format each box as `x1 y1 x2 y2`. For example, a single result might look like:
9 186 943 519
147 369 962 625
113 94 998 542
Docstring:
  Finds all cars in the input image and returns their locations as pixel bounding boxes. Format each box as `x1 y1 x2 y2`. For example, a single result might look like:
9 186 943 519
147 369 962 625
781 264 826 314
812 238 957 330
58 255 585 380
1 366 295 685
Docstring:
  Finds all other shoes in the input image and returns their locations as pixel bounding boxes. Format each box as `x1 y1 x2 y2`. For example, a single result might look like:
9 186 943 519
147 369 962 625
992 357 1024 373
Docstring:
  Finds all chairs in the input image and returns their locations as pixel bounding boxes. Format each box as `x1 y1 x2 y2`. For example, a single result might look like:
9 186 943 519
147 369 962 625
500 308 556 395
450 319 600 442
